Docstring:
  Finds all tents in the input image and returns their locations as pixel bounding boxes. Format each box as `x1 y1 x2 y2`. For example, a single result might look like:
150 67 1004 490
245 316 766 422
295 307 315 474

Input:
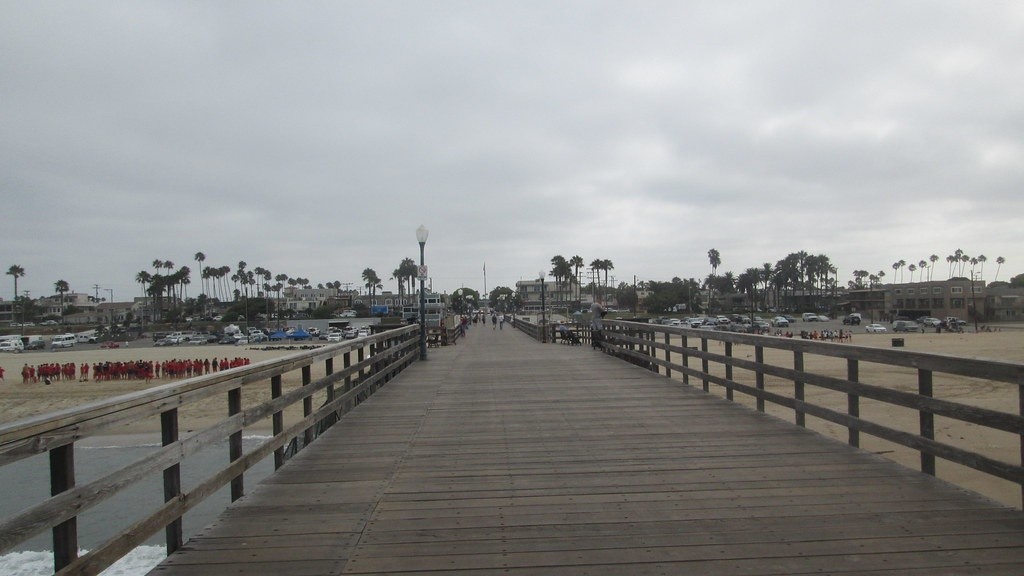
270 329 312 343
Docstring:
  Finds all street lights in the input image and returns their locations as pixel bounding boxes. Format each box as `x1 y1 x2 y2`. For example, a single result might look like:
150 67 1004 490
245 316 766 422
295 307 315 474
417 225 430 361
539 269 547 344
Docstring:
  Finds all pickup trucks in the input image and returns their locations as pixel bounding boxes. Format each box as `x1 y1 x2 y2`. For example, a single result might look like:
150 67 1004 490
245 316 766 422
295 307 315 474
944 317 967 326
76 334 98 344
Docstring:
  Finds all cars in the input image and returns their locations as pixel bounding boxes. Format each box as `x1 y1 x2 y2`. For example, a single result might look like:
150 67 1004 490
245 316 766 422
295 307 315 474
892 321 918 333
213 316 222 321
0 336 47 354
802 313 818 323
916 316 930 324
341 310 357 318
153 324 369 347
782 315 797 323
771 317 789 327
924 318 941 328
100 341 120 349
842 316 861 326
865 323 887 334
818 316 830 322
668 313 770 333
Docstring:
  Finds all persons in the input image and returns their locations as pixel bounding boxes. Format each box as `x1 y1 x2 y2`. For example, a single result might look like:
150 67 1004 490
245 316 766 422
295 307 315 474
591 296 606 330
560 320 569 337
461 310 504 337
22 358 249 384
0 367 5 381
774 329 852 344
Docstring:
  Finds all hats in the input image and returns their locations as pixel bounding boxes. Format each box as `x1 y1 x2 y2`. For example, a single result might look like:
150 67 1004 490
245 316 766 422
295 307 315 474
559 320 565 324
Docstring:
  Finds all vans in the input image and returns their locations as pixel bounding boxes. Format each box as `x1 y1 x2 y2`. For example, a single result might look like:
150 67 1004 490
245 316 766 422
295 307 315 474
50 334 76 348
